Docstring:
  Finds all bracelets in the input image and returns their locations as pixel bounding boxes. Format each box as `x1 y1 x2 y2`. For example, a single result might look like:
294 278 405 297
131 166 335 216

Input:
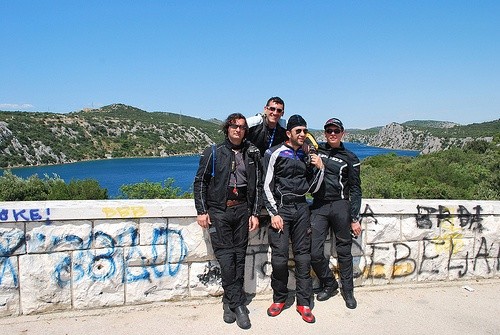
253 214 259 217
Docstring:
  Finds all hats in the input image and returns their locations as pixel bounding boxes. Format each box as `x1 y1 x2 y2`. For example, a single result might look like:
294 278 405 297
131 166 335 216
324 118 343 130
287 114 307 130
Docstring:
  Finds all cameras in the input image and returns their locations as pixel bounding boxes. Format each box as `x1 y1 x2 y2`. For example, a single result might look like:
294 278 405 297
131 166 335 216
350 229 358 239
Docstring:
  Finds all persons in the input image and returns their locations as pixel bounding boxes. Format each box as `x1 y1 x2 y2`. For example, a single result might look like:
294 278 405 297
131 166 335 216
261 114 362 324
245 96 289 157
194 112 261 329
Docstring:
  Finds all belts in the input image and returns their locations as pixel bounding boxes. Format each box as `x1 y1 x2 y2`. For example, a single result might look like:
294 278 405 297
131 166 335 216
226 198 247 206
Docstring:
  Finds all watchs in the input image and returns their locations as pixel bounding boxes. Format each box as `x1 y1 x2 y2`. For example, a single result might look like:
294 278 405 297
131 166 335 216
351 218 358 223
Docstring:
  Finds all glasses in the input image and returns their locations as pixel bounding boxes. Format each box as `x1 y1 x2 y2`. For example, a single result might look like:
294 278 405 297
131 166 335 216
229 123 247 130
296 129 308 134
326 128 342 134
266 105 284 114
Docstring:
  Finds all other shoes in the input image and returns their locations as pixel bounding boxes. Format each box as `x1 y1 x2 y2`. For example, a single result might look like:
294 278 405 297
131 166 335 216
317 282 339 301
223 303 251 330
267 299 286 316
341 288 357 309
295 304 315 323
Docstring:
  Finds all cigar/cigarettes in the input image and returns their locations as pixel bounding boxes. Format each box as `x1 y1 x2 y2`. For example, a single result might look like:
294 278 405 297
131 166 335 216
278 229 281 233
